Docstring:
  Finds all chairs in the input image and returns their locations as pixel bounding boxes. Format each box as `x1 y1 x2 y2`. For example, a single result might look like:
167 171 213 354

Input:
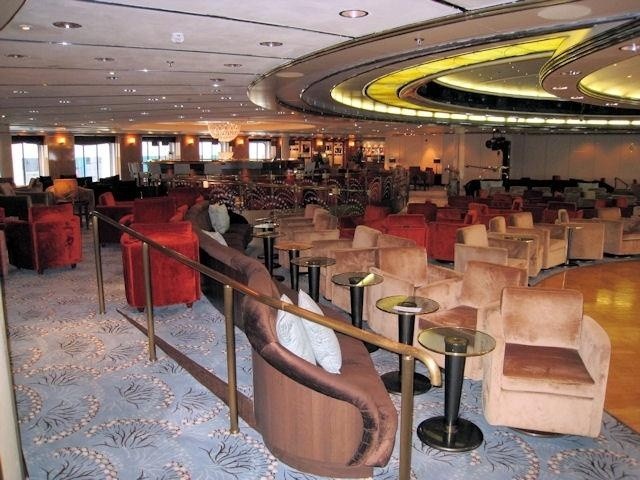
1 160 222 316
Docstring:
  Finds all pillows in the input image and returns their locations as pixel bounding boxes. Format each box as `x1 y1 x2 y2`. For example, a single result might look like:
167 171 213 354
275 292 317 368
295 289 342 375
203 201 231 248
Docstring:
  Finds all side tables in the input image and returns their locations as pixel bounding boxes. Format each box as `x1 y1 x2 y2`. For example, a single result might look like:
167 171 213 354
330 271 384 352
420 326 496 452
375 296 439 396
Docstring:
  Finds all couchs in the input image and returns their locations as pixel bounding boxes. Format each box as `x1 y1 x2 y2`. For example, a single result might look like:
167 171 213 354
415 261 527 381
259 164 638 322
180 195 396 479
482 287 611 439
